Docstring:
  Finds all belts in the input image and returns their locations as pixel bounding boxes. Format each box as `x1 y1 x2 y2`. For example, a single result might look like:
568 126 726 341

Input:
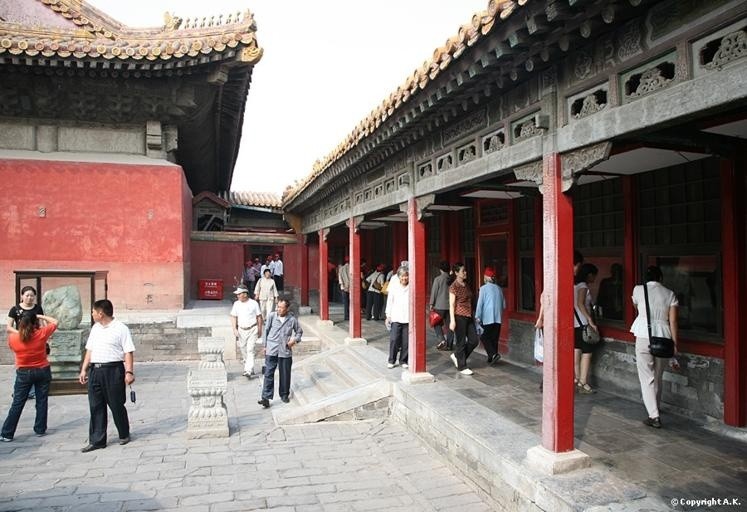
89 362 122 368
240 323 257 330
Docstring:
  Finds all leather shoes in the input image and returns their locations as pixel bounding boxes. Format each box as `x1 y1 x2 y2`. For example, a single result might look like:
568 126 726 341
258 399 270 407
120 437 130 445
80 443 106 452
281 397 289 402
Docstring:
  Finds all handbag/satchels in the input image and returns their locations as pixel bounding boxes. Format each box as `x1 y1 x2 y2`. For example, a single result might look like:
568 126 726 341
583 323 601 345
649 337 674 358
372 280 390 295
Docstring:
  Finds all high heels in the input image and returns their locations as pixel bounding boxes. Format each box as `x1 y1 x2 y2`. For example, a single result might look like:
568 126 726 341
573 377 597 394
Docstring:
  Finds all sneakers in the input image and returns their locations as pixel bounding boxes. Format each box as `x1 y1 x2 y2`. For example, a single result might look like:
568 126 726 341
488 353 500 365
0 432 12 442
449 352 474 375
243 372 251 376
399 364 408 369
642 417 662 428
388 364 394 368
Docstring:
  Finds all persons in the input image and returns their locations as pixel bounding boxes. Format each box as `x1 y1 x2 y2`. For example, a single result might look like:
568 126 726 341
242 252 409 325
258 296 302 408
628 266 679 428
572 264 599 394
78 299 136 453
6 286 50 398
429 260 506 375
0 310 58 442
594 262 624 317
384 266 408 369
230 284 263 377
534 250 584 393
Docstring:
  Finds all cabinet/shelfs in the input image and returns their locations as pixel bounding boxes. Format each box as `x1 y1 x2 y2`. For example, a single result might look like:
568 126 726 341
10 265 110 400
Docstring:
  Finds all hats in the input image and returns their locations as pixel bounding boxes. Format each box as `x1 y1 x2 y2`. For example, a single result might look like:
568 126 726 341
233 287 249 295
484 267 496 277
